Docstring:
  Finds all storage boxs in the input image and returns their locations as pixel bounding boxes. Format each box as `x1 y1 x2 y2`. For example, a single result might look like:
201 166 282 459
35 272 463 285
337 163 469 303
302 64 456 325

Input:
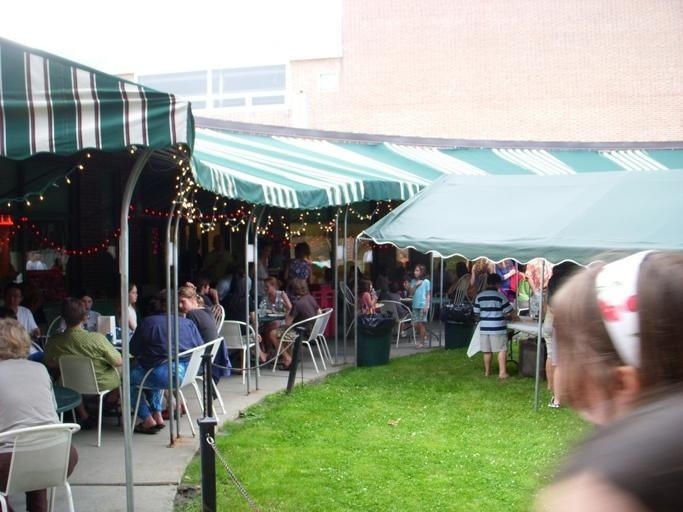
519 338 546 378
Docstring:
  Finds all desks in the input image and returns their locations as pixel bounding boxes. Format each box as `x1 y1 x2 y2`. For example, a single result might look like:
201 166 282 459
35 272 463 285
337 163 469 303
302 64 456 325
505 321 543 369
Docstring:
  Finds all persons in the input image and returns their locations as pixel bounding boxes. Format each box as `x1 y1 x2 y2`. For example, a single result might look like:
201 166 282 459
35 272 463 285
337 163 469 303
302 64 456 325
532 250 683 512
403 247 586 408
0 234 412 512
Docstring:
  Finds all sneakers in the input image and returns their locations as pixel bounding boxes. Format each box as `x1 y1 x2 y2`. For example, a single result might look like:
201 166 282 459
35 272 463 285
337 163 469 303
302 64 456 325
78 415 98 430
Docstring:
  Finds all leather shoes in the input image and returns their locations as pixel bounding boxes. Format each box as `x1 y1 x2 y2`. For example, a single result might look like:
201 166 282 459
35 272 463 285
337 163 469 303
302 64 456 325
161 409 182 421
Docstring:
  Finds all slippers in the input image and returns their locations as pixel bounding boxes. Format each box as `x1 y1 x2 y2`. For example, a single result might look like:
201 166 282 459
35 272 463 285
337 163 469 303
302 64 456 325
279 361 292 371
548 396 560 408
155 422 166 431
133 424 160 435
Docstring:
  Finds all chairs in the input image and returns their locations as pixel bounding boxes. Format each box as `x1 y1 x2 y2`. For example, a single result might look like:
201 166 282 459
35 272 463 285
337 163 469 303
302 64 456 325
1 282 335 512
338 283 417 364
477 272 487 290
451 273 472 304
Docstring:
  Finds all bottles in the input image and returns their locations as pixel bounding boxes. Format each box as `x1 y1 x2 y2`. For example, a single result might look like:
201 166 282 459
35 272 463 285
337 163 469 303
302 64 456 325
370 288 377 302
82 312 90 326
258 297 285 319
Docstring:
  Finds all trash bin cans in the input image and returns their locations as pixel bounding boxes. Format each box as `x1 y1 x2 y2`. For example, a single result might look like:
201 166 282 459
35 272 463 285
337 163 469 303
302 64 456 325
445 317 474 349
358 314 393 366
